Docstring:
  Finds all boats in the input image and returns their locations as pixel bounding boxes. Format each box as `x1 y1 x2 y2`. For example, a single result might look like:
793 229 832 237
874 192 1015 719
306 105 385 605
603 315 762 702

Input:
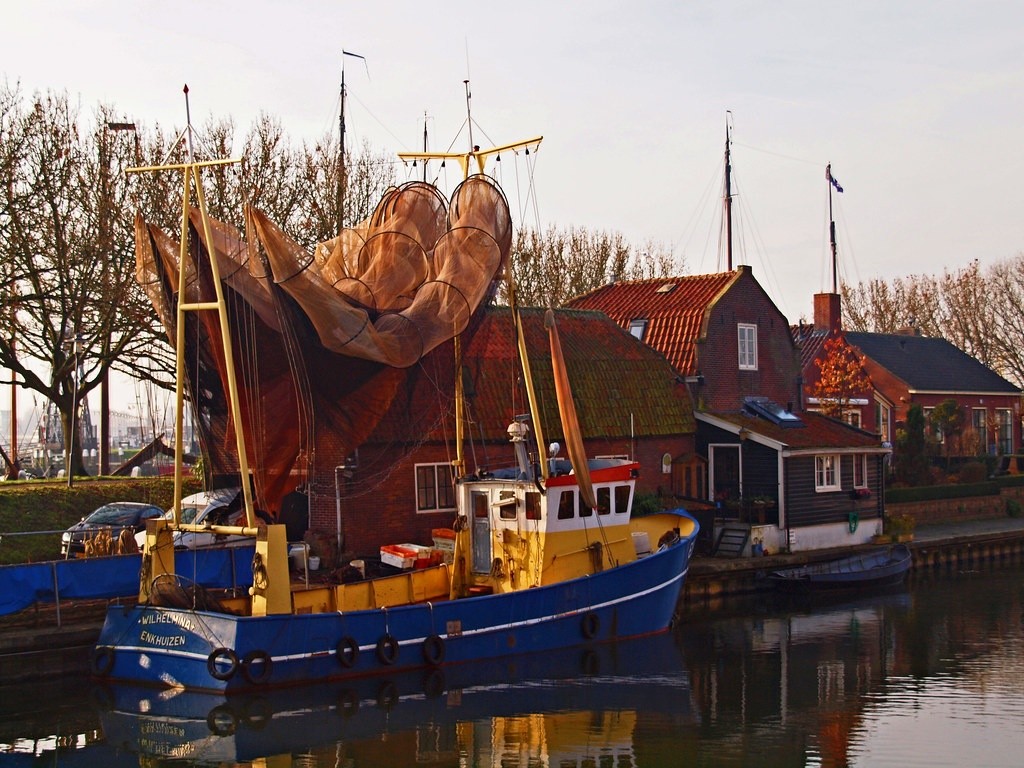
99 629 689 768
768 545 913 585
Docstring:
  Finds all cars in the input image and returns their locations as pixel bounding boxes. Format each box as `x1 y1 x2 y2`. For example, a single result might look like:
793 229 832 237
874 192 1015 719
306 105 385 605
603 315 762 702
61 488 266 559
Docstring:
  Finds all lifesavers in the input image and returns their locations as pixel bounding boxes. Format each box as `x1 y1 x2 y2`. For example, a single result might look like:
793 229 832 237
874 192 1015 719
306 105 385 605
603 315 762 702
377 634 400 663
580 610 602 637
245 700 274 731
420 668 445 696
242 649 273 685
337 636 360 669
377 686 400 712
88 686 117 713
420 634 447 665
205 647 238 680
207 706 240 738
89 647 115 676
337 691 363 718
582 650 603 676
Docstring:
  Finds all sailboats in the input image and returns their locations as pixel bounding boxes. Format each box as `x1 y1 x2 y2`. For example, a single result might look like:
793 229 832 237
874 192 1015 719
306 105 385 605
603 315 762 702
92 51 699 695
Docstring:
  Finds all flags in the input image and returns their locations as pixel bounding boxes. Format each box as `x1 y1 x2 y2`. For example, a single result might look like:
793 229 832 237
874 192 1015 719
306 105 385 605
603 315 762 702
830 175 843 192
